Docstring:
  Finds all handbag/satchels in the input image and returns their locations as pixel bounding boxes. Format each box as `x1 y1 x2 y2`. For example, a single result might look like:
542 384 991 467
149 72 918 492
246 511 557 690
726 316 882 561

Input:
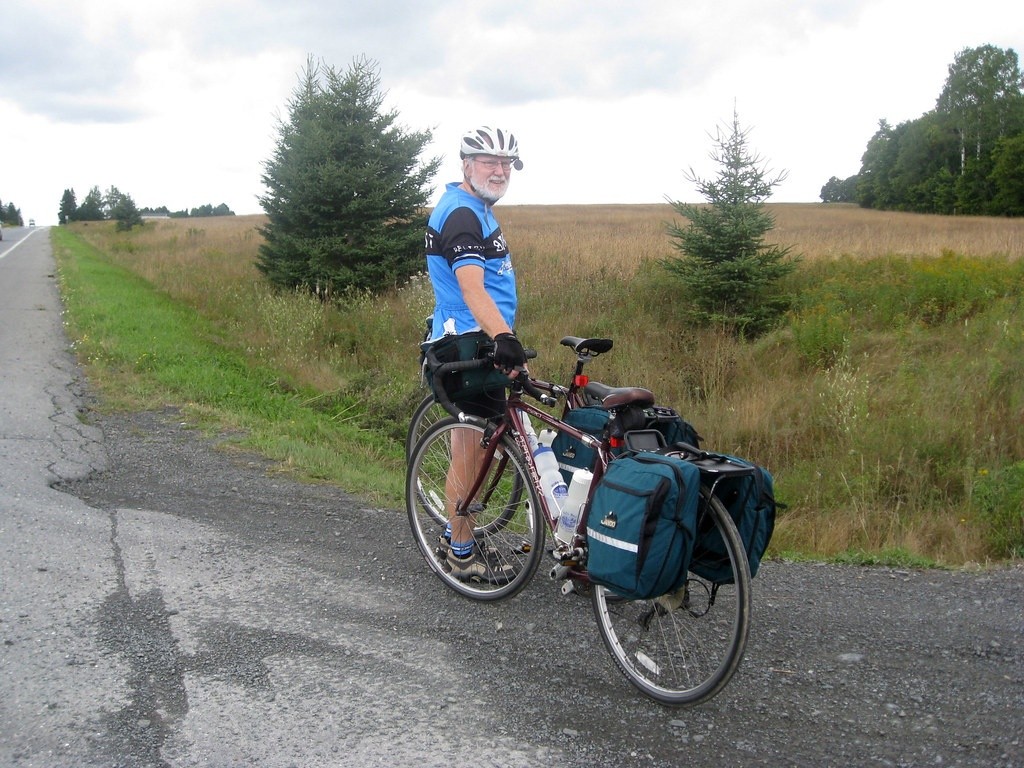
646 406 701 449
586 448 701 600
688 451 776 585
551 403 624 488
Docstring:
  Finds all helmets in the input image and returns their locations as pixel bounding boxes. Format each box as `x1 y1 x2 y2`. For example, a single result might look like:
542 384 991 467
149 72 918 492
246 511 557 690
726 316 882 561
460 124 519 157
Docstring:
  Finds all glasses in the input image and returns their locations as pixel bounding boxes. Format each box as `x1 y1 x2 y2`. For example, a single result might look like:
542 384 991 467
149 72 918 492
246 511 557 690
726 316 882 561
472 158 514 170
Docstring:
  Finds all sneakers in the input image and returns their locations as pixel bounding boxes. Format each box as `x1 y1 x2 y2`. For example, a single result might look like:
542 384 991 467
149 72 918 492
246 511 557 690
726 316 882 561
443 539 519 585
434 531 502 561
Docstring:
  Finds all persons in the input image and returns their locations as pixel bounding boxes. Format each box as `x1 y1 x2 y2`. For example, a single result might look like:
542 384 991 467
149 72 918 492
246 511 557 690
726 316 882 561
422 121 531 585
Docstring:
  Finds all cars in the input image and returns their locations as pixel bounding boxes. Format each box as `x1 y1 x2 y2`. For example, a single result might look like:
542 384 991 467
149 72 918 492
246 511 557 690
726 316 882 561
0 221 3 240
29 218 35 226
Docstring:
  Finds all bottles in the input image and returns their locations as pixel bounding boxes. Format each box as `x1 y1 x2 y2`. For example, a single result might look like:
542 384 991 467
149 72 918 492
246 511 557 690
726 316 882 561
558 467 593 544
533 443 568 518
536 428 556 447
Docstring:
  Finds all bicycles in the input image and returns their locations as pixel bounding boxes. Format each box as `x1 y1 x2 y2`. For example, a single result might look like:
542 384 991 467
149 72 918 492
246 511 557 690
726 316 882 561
404 344 753 707
407 335 680 537
